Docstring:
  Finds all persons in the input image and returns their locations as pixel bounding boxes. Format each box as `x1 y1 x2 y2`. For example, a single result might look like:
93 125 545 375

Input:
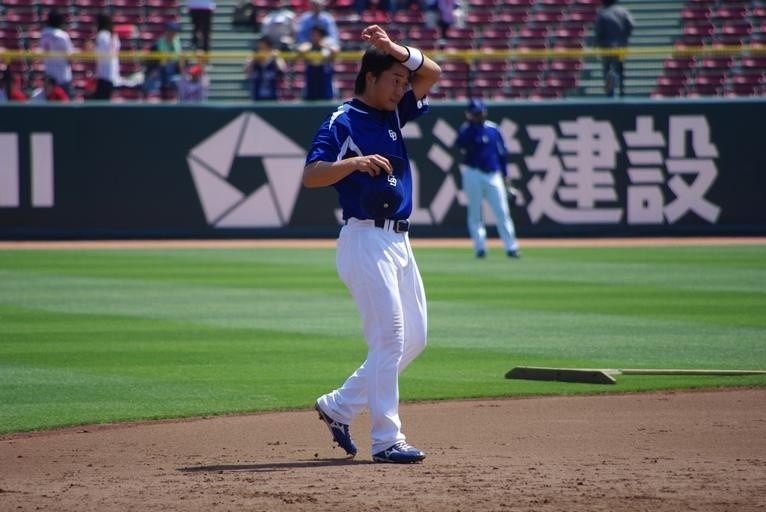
0 0 216 101
233 0 464 101
592 0 637 97
453 98 523 259
303 24 442 463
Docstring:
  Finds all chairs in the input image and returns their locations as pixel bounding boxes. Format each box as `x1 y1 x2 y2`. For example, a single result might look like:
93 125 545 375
252 0 601 104
2 0 181 101
644 0 765 97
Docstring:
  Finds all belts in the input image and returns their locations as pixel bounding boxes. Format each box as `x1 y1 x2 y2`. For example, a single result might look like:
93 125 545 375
374 218 411 233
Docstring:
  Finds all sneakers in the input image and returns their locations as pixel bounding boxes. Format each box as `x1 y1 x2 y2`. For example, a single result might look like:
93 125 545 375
478 250 485 256
315 401 357 455
373 441 425 464
510 249 520 257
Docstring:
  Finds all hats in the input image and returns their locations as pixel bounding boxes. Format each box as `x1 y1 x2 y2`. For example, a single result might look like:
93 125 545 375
466 97 488 113
